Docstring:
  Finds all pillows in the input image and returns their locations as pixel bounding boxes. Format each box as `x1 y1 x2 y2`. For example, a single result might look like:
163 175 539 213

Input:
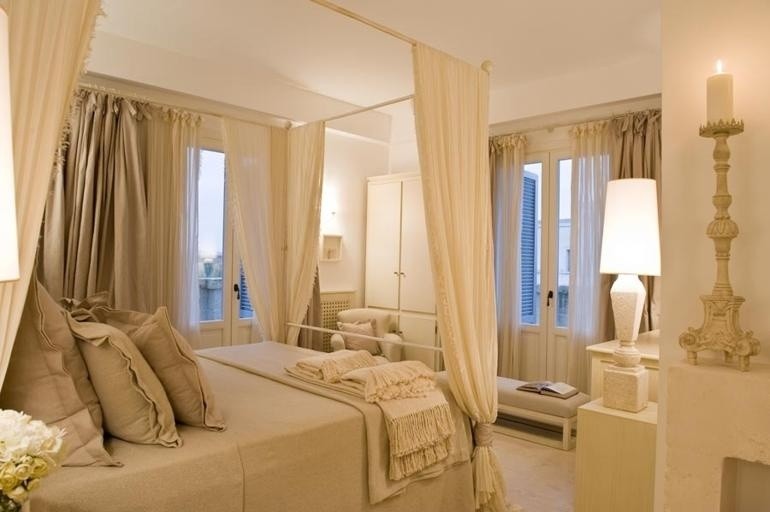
336 321 381 354
102 308 227 433
0 245 123 468
69 311 181 449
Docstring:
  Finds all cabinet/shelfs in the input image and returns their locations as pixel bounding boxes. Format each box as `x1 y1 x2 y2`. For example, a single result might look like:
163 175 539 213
370 307 444 371
364 178 436 316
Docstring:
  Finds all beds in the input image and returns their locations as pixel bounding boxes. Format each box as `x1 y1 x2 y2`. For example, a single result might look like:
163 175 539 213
0 242 479 512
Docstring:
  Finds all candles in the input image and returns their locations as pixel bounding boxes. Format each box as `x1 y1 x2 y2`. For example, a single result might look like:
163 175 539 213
706 58 733 125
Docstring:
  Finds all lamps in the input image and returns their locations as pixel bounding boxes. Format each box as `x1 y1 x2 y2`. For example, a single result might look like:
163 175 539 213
599 178 662 413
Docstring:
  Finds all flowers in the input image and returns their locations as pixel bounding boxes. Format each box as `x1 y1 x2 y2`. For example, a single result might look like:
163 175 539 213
0 408 68 512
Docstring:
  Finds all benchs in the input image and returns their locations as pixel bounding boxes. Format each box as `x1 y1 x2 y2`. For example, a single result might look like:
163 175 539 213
438 370 590 450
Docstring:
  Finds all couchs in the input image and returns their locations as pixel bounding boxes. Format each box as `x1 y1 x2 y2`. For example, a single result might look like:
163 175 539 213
330 309 403 363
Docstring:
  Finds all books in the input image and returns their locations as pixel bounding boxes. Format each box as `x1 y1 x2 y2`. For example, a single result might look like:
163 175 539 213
516 381 580 399
